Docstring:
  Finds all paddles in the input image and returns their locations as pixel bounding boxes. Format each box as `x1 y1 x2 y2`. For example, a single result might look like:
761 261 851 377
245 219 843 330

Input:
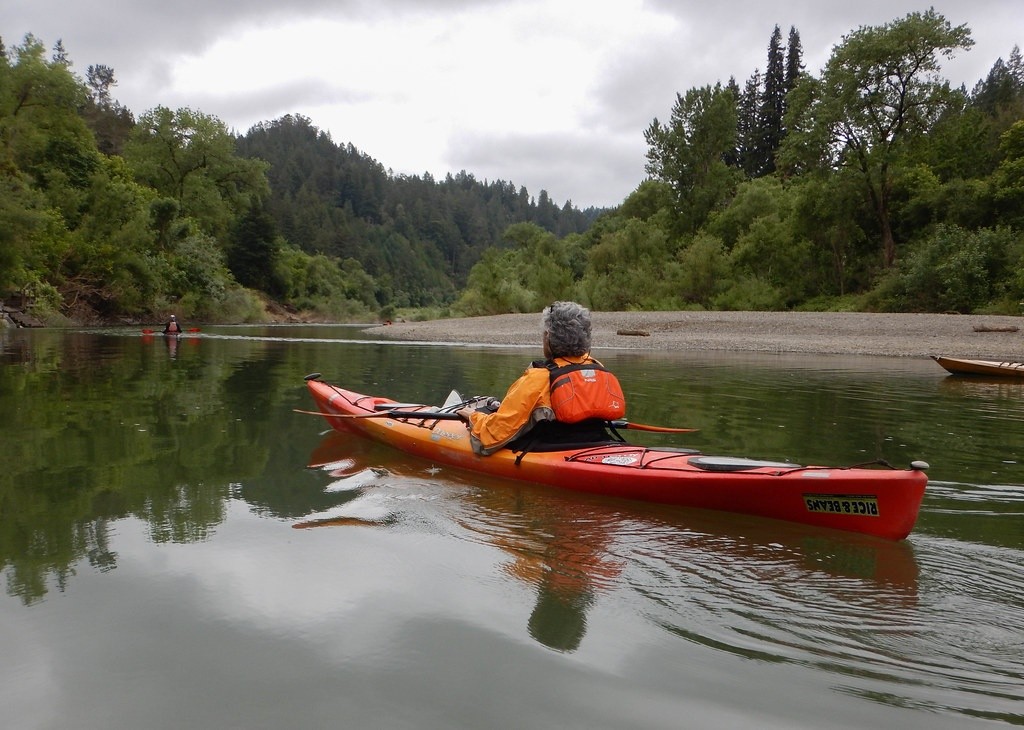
140 326 201 334
292 404 699 432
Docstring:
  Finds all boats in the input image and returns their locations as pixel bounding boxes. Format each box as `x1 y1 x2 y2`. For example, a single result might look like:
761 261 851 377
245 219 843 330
929 354 1024 378
306 379 928 542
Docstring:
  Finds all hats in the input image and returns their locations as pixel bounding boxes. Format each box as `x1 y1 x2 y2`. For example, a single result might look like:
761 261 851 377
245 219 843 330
171 315 175 318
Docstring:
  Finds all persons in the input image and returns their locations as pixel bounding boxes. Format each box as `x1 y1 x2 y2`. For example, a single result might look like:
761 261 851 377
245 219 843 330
456 301 625 457
162 314 182 334
